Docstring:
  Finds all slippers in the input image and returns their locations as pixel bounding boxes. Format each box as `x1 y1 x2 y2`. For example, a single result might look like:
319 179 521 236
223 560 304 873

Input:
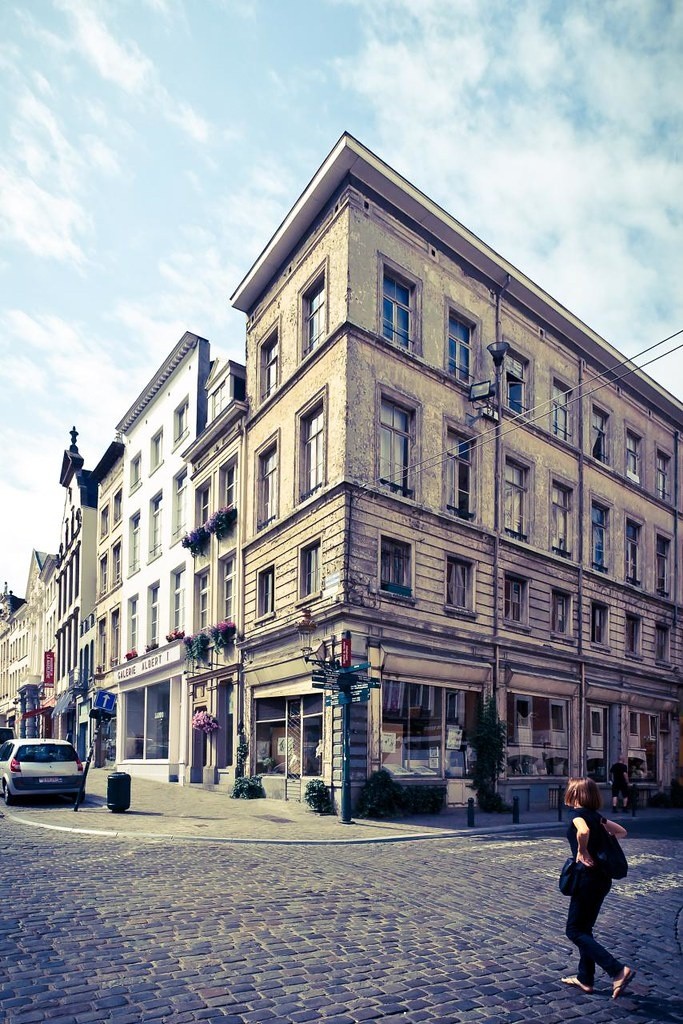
613 971 637 1000
561 976 594 995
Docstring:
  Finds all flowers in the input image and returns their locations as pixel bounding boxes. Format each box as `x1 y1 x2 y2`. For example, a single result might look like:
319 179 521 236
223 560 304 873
185 619 238 666
191 708 225 735
184 505 239 555
119 628 185 661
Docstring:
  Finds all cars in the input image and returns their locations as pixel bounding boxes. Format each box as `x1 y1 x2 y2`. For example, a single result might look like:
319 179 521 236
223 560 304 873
0 739 85 806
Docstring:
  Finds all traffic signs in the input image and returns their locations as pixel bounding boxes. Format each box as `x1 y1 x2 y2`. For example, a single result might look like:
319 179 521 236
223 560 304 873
311 662 381 706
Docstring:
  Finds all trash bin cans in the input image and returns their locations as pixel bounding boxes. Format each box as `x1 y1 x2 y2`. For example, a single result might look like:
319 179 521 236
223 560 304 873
106 772 131 813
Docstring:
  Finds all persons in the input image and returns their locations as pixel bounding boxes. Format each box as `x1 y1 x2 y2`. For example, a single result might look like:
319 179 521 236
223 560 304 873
608 753 629 814
559 777 638 1001
315 740 321 759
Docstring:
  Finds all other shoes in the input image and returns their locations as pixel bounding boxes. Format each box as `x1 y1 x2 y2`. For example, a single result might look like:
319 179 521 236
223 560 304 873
612 806 618 813
622 807 630 813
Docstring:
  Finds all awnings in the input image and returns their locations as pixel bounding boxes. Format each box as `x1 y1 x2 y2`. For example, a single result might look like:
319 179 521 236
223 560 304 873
21 706 54 720
50 694 75 720
4 716 14 723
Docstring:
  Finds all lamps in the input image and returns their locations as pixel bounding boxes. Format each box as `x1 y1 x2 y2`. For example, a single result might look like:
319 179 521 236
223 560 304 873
291 607 326 667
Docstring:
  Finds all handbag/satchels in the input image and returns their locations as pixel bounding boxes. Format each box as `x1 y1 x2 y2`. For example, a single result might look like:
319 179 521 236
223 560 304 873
605 833 628 880
558 857 584 897
